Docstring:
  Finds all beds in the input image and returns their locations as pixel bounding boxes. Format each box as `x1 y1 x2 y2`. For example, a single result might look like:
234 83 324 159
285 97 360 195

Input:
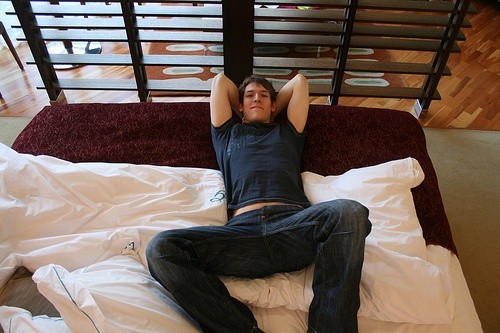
0 102 483 333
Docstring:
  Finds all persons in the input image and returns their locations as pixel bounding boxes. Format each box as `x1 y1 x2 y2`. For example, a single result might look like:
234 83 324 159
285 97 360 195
145 73 372 333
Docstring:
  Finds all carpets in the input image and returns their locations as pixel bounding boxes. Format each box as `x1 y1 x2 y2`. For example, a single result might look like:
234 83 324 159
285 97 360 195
145 0 409 96
